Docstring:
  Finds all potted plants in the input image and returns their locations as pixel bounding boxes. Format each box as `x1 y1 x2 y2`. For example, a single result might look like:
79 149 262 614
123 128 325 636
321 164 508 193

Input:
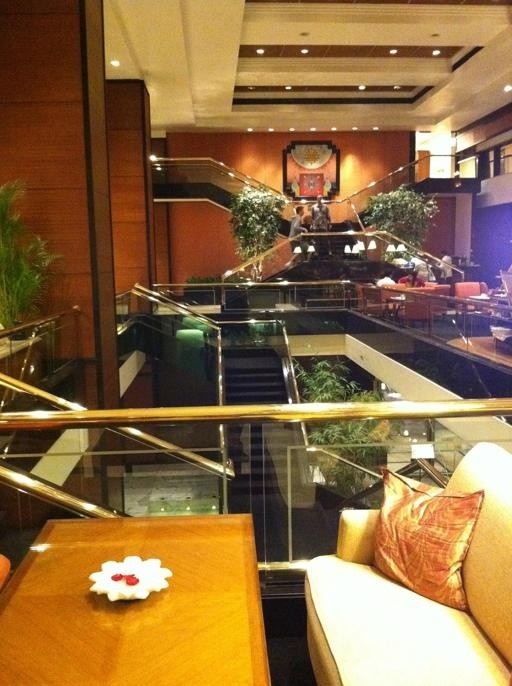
1 178 59 340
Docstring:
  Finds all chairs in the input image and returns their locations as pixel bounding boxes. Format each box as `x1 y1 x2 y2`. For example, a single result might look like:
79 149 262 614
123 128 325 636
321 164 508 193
357 279 491 331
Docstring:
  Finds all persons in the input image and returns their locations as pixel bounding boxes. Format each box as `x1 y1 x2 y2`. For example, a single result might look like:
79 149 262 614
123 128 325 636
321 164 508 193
283 206 307 268
386 250 455 308
291 174 331 197
308 194 333 256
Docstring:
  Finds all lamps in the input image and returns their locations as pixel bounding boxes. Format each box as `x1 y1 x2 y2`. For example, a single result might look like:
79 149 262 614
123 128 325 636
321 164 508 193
343 235 378 254
293 236 315 255
385 241 407 253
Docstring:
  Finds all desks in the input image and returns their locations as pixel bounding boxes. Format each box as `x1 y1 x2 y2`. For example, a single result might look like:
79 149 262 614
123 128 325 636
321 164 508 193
0 511 272 686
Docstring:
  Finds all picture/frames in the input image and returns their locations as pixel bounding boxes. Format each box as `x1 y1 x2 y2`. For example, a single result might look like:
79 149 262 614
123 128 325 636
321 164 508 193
282 140 341 203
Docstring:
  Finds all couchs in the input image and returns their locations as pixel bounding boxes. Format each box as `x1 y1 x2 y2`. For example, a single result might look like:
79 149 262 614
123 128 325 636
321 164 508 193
298 439 512 684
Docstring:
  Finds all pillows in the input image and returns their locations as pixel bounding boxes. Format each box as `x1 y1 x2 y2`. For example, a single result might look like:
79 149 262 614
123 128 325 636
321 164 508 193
367 464 486 615
